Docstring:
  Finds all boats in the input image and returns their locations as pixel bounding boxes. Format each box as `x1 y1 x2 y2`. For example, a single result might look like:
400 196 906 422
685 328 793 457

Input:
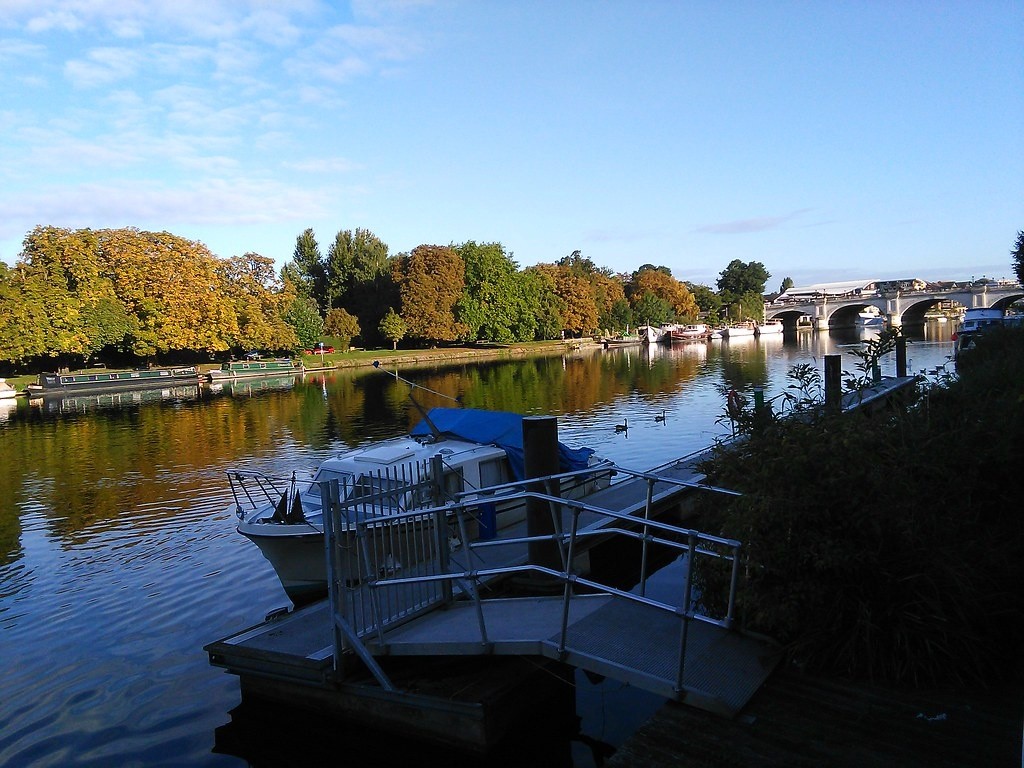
0 378 17 399
952 307 1024 350
27 358 298 395
602 317 784 347
225 359 620 598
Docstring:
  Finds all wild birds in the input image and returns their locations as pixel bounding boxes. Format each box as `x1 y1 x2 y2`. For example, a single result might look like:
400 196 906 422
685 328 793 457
614 418 629 429
656 410 666 420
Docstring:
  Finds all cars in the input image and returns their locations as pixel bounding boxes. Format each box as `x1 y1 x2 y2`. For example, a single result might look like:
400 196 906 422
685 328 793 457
300 349 312 355
313 346 335 355
243 350 265 359
